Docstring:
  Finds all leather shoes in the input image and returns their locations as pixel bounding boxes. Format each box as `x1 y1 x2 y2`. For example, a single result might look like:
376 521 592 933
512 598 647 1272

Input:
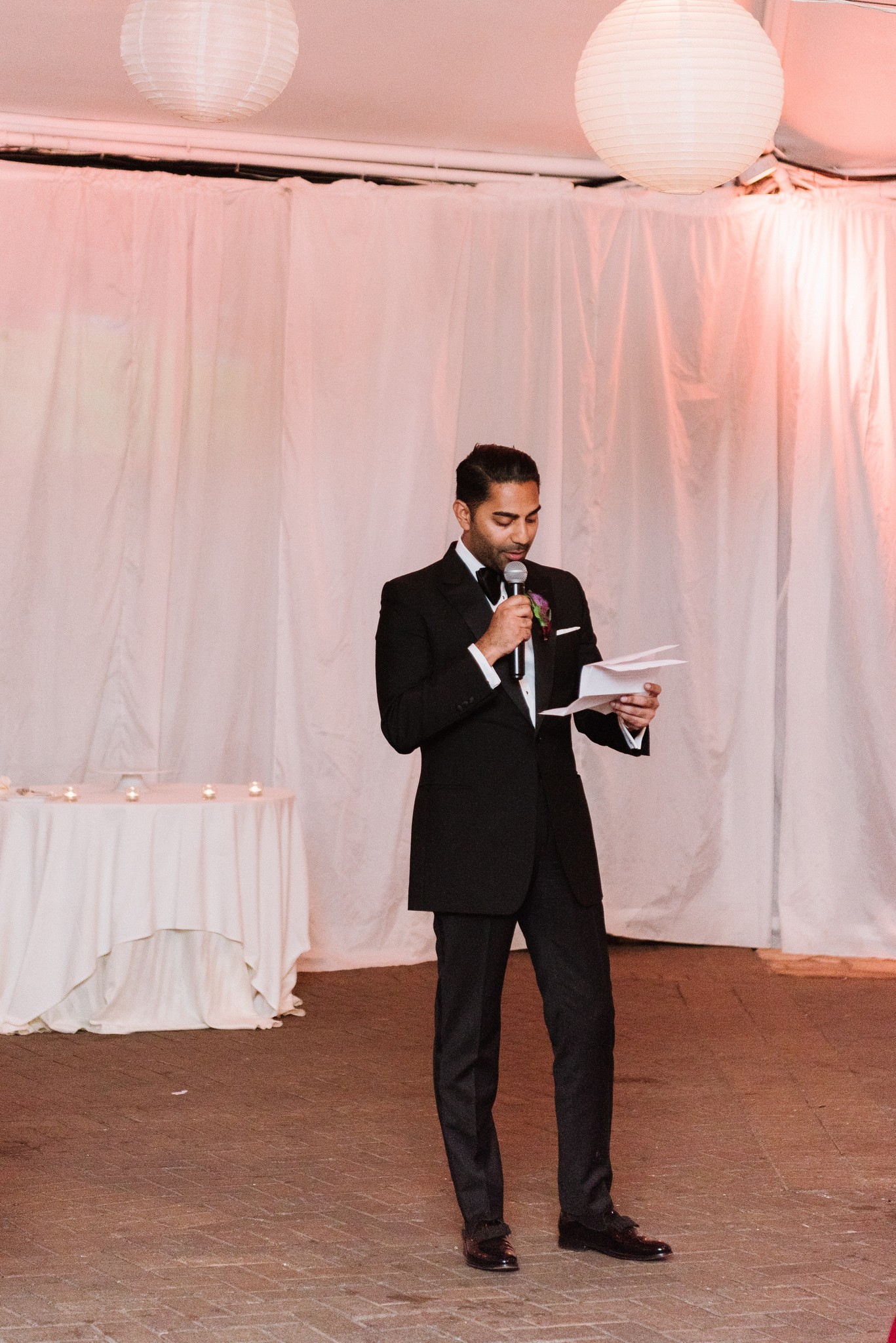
462 1228 519 1271
559 1207 674 1260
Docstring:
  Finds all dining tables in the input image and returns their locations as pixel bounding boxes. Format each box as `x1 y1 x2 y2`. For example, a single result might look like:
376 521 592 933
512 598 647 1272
0 782 313 1035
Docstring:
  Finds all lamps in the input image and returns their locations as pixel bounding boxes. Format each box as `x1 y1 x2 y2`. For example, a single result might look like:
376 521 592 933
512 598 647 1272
739 156 780 186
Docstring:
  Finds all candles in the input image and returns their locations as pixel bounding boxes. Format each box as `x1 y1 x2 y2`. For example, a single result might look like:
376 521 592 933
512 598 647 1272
64 787 77 802
202 784 216 800
125 786 139 802
248 783 265 798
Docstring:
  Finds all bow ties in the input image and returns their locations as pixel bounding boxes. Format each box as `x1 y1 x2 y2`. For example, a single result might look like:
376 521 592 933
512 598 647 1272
475 565 515 605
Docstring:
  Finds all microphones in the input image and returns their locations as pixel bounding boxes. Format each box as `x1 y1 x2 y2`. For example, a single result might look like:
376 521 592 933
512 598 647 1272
503 561 528 680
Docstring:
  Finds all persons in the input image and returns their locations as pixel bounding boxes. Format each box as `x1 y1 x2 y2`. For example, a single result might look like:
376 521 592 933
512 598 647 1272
371 439 672 1274
